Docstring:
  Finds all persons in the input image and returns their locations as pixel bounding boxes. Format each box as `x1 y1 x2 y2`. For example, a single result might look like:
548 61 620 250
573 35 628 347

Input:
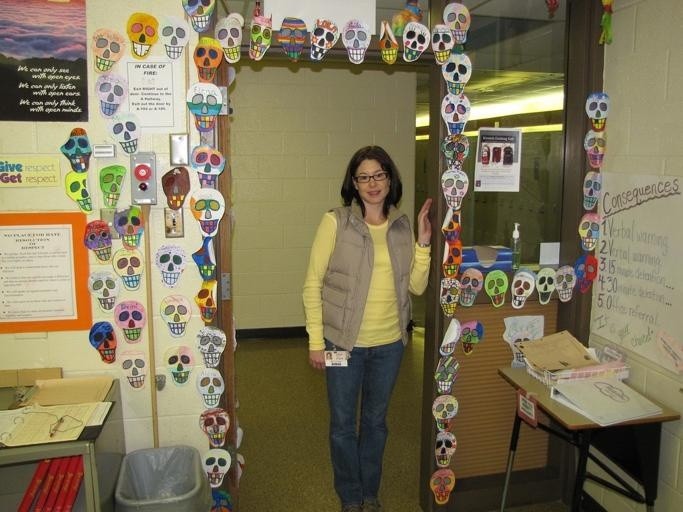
325 352 332 360
301 144 433 510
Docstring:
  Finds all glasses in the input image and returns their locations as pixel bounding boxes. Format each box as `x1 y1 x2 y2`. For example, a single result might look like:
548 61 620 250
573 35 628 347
352 172 389 184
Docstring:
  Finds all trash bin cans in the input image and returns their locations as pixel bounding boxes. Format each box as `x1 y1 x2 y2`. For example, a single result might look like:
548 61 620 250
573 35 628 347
114 444 209 512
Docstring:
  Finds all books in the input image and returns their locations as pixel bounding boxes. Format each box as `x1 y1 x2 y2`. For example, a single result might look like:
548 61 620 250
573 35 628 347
516 330 661 427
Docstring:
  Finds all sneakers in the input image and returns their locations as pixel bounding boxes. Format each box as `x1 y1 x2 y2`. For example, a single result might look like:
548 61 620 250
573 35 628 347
342 498 383 511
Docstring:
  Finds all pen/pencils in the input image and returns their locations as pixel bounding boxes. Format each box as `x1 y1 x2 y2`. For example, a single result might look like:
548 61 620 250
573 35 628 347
49 416 63 437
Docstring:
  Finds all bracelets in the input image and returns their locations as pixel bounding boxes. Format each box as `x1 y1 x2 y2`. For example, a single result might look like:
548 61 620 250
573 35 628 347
415 242 434 248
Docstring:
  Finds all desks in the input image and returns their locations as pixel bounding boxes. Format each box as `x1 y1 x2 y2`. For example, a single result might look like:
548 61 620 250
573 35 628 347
498 366 680 512
0 378 125 512
